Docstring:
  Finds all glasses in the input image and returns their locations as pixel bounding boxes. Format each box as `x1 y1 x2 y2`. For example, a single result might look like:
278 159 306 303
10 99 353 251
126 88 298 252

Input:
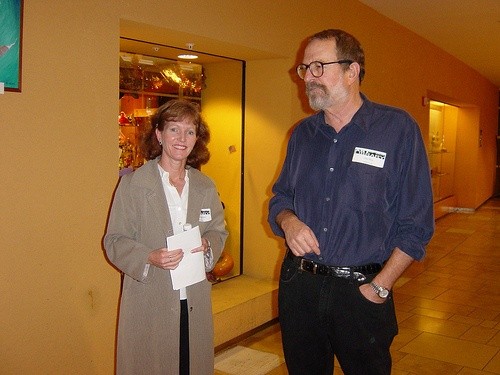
296 60 351 79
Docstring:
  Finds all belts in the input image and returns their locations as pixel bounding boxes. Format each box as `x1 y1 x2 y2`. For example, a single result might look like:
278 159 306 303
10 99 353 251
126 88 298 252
287 251 381 282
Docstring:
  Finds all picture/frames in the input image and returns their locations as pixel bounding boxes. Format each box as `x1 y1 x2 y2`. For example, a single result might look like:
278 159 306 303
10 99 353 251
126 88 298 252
0 0 24 92
